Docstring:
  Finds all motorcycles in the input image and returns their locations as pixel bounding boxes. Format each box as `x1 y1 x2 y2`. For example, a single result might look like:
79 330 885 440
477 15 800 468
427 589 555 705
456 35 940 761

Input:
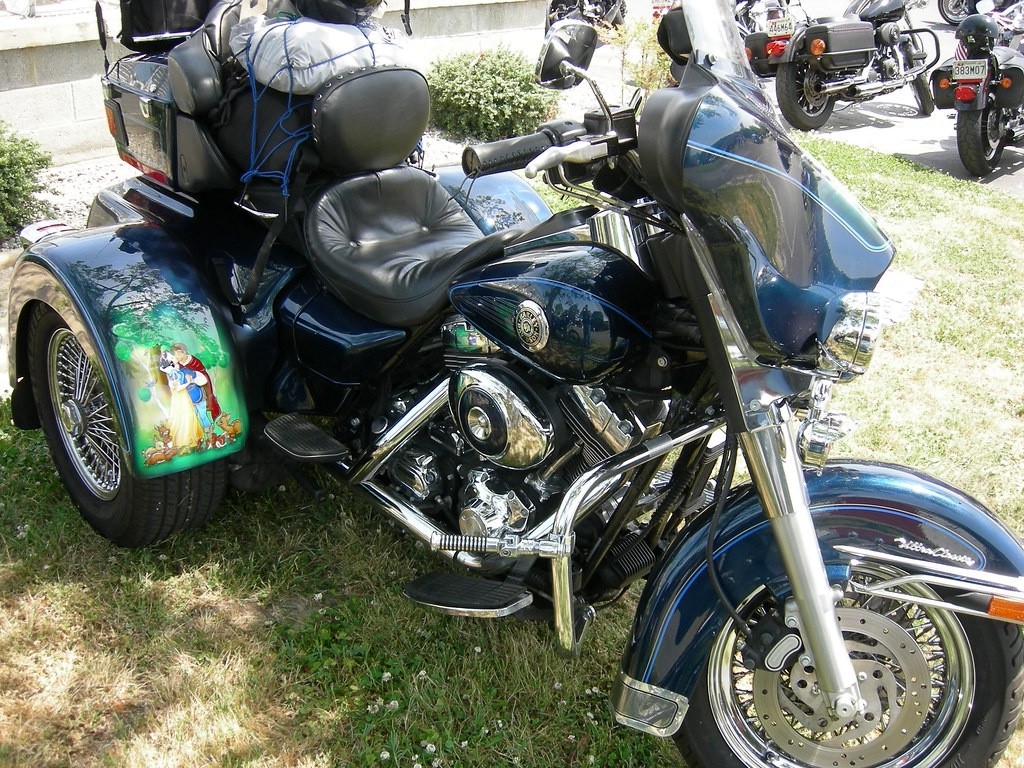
0 0 1024 768
542 0 628 52
769 0 943 131
928 0 1024 177
726 0 821 80
